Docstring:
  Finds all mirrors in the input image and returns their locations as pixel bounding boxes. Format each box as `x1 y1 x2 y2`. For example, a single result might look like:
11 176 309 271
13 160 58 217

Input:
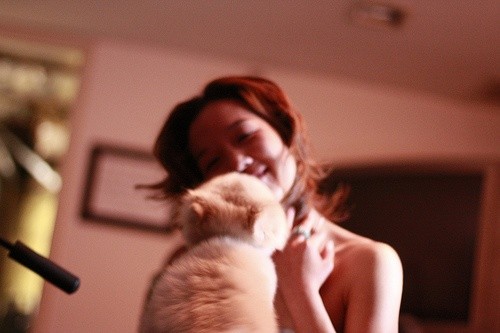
0 31 86 333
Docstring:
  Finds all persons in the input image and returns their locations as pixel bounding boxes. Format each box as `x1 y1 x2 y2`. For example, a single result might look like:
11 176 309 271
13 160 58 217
133 74 403 333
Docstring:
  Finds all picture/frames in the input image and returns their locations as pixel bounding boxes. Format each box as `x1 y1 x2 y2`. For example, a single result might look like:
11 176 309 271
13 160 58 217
79 140 180 236
322 149 500 333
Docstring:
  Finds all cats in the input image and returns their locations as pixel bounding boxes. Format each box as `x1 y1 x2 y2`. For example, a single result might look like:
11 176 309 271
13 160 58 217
139 171 287 333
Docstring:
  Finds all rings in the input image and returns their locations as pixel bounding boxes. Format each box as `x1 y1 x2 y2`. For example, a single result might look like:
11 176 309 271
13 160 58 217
292 226 309 238
